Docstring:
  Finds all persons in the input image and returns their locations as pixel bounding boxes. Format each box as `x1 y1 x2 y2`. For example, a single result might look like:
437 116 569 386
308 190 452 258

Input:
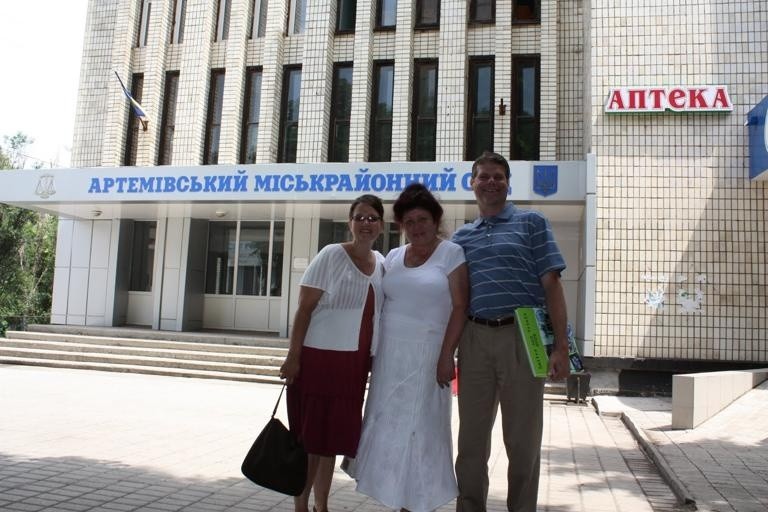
339 181 470 511
276 192 388 511
447 147 572 512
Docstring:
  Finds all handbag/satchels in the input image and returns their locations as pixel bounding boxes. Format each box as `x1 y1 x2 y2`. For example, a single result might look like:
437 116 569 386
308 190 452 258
241 383 308 497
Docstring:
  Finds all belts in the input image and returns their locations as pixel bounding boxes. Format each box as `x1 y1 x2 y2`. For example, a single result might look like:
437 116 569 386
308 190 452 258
467 315 515 328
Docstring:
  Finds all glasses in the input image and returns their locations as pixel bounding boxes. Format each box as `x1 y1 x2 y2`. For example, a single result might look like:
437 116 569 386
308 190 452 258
352 214 381 222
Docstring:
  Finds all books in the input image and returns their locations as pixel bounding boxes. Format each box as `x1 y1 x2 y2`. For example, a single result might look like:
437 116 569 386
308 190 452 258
515 306 587 377
